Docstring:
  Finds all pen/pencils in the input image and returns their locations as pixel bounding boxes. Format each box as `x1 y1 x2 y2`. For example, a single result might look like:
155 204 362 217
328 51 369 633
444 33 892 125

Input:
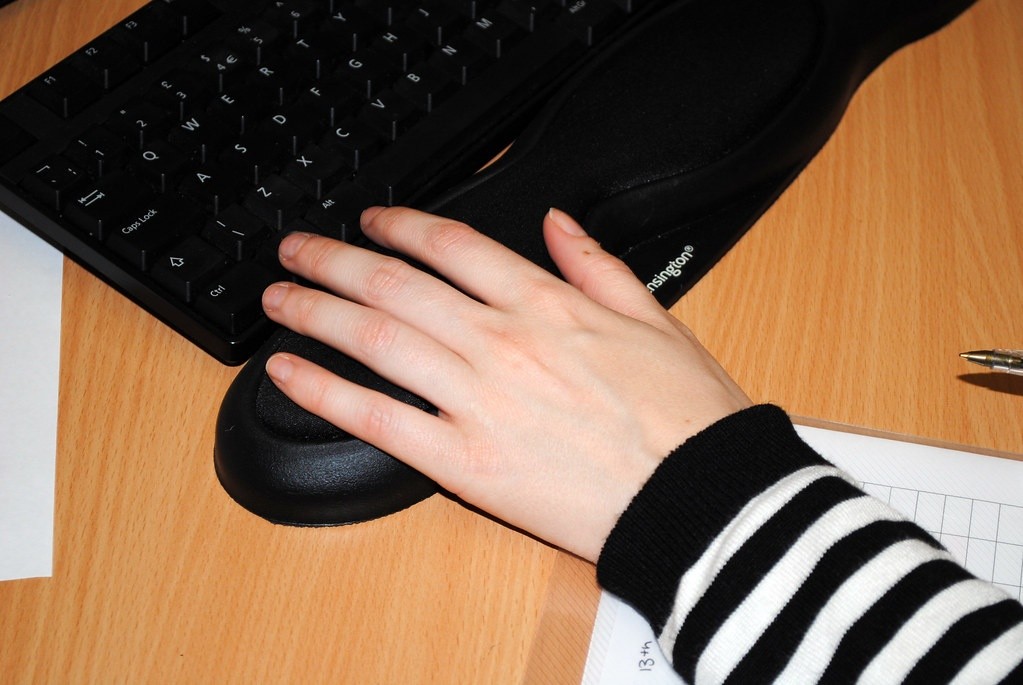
959 349 1021 378
958 372 1023 395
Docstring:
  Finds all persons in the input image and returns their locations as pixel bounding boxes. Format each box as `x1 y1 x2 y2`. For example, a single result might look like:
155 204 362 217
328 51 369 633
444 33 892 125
262 205 1023 685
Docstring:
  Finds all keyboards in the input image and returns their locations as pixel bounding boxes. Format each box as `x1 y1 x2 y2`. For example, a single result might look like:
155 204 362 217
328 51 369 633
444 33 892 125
0 0 684 366
